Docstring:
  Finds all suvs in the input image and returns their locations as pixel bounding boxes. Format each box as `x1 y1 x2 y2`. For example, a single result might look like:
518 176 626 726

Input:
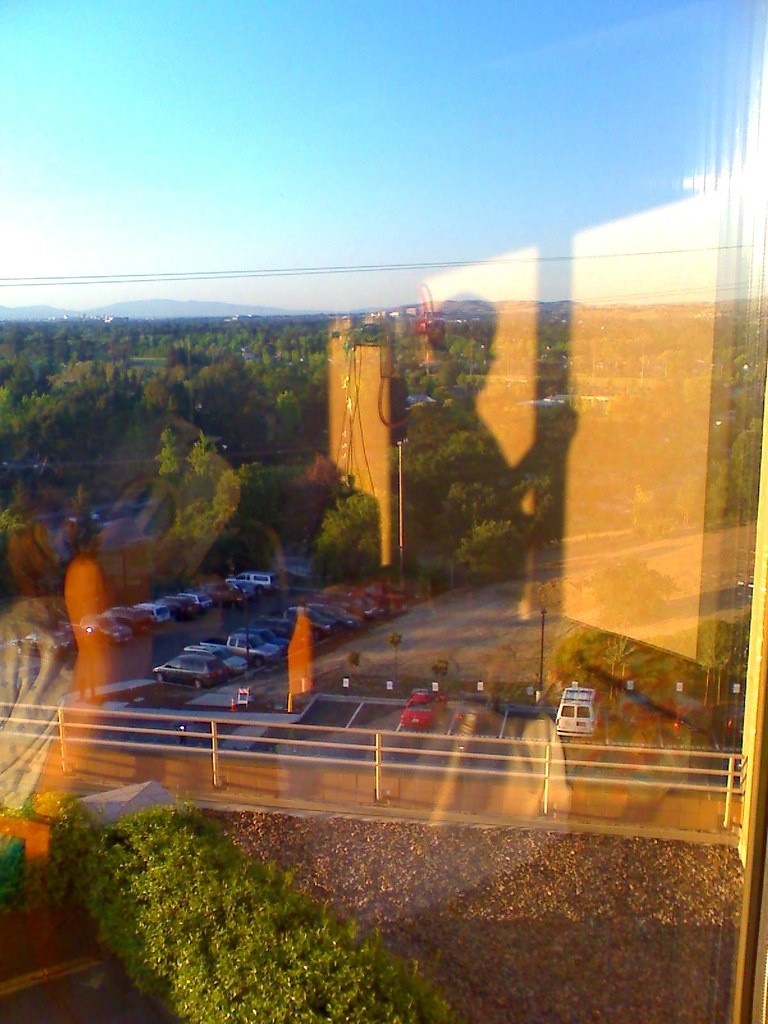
152 579 405 687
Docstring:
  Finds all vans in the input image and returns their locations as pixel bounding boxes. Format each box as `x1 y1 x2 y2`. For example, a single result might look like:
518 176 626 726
553 686 602 739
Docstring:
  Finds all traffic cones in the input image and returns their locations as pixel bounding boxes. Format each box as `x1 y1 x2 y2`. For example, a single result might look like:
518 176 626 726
230 698 237 713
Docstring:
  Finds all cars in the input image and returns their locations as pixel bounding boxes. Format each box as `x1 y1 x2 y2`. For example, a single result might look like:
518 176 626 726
1 574 283 657
400 688 448 730
